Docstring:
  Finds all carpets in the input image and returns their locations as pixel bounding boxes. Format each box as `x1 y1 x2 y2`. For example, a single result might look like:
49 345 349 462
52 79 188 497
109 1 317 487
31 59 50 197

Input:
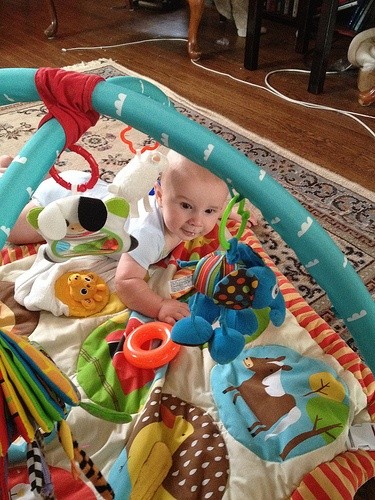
2 60 375 374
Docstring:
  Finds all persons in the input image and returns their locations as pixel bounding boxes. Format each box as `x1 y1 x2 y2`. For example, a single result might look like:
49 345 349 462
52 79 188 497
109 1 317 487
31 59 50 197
0 154 259 324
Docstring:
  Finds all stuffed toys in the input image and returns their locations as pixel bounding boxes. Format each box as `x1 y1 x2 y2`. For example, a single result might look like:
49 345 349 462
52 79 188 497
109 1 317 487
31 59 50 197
108 147 174 218
171 241 285 364
56 269 110 317
